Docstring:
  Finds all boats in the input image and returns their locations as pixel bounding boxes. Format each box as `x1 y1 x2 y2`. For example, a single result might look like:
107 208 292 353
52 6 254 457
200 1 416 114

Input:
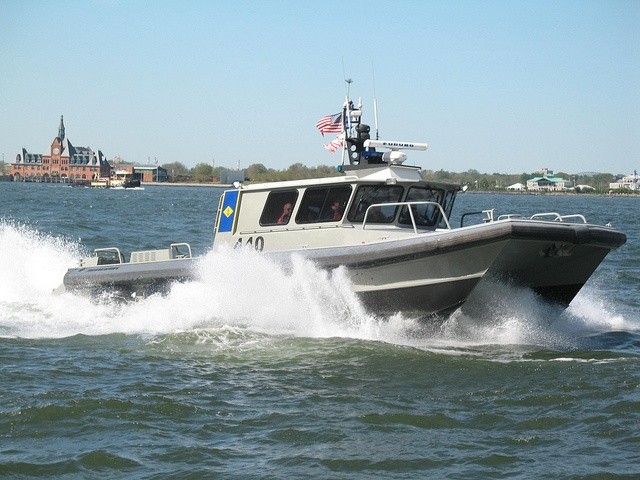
67 177 140 189
63 58 628 341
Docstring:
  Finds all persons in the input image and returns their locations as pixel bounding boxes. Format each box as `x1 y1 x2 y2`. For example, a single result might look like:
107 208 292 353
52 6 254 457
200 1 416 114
277 203 293 224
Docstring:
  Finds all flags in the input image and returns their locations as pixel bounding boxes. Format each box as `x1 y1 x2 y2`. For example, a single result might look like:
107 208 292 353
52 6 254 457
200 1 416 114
315 111 344 136
323 133 347 153
